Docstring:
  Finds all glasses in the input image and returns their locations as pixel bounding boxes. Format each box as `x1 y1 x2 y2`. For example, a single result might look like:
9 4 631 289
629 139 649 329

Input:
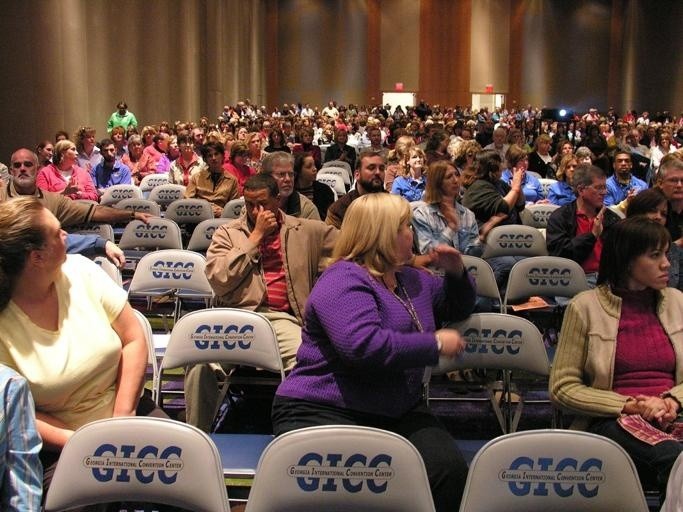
12 161 34 168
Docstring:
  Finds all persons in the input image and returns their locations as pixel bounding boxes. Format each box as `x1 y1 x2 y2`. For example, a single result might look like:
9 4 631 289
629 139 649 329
0 97 683 512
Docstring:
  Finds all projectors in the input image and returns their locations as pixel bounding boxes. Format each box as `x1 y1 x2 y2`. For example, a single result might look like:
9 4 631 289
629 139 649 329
542 107 573 121
